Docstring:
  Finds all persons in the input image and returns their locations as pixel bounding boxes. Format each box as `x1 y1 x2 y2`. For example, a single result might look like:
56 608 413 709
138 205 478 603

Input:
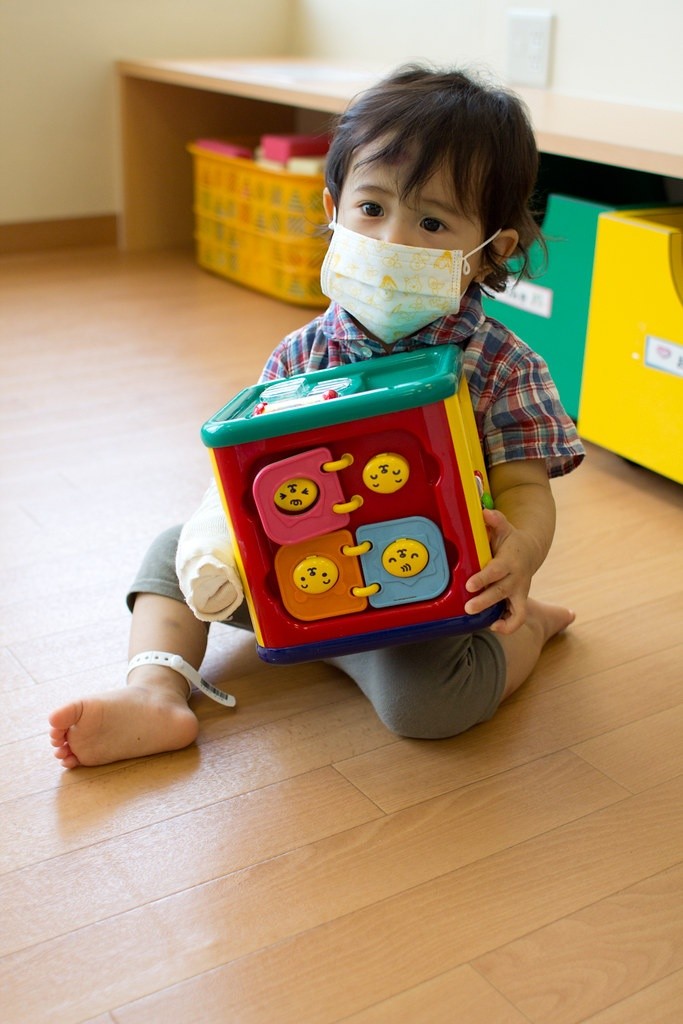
48 57 586 769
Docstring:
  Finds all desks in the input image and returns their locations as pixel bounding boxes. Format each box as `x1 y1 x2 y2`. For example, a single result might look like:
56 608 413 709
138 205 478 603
110 53 683 256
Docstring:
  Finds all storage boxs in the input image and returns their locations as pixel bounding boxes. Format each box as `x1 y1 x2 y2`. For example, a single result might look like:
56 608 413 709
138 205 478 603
185 135 331 310
574 206 682 487
480 190 683 419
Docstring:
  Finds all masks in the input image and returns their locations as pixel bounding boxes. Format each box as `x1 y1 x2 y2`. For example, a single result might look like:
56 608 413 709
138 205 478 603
320 205 492 344
